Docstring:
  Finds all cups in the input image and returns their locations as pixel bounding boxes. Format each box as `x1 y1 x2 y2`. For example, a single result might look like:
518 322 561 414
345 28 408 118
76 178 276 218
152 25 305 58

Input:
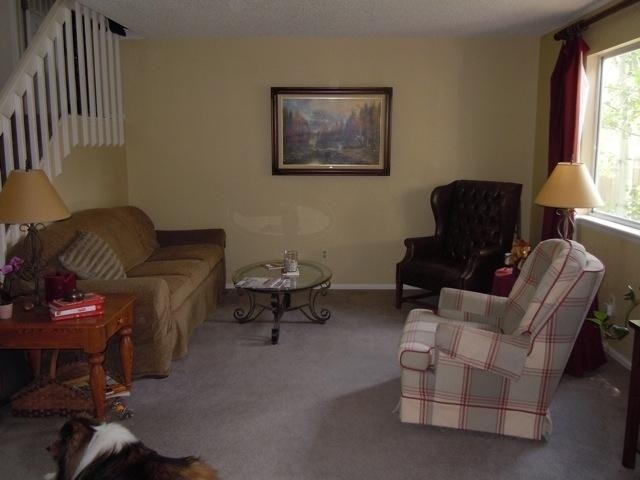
283 248 299 273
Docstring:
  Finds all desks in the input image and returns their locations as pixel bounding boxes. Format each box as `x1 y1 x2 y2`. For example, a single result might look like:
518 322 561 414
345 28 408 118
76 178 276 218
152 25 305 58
490 259 609 381
1 292 138 423
620 317 640 472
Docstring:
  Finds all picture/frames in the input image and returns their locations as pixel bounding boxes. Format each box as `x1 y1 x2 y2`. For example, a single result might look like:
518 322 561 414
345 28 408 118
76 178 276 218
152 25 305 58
269 84 394 179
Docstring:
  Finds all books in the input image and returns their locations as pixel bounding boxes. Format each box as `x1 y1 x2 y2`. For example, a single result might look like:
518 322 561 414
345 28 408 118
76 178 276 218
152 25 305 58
63 373 130 402
234 261 291 290
47 292 106 323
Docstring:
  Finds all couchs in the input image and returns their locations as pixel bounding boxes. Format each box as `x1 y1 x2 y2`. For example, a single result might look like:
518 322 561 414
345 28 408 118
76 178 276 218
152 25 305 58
393 176 524 310
6 205 231 379
391 237 611 448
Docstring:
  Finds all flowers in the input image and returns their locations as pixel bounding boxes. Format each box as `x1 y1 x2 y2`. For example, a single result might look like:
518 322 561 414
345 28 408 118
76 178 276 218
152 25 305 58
0 256 27 306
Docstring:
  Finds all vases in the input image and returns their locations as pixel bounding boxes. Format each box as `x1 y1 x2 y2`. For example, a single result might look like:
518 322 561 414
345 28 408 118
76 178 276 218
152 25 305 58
0 304 15 320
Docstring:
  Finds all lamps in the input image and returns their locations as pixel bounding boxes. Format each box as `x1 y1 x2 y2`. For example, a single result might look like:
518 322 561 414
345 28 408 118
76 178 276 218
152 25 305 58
536 157 603 236
1 166 71 308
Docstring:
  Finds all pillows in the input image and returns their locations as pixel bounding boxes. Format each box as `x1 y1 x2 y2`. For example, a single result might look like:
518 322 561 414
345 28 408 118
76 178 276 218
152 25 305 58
56 229 130 285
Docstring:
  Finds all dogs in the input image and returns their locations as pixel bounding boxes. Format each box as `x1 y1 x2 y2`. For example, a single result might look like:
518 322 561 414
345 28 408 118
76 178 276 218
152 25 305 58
42 412 224 480
